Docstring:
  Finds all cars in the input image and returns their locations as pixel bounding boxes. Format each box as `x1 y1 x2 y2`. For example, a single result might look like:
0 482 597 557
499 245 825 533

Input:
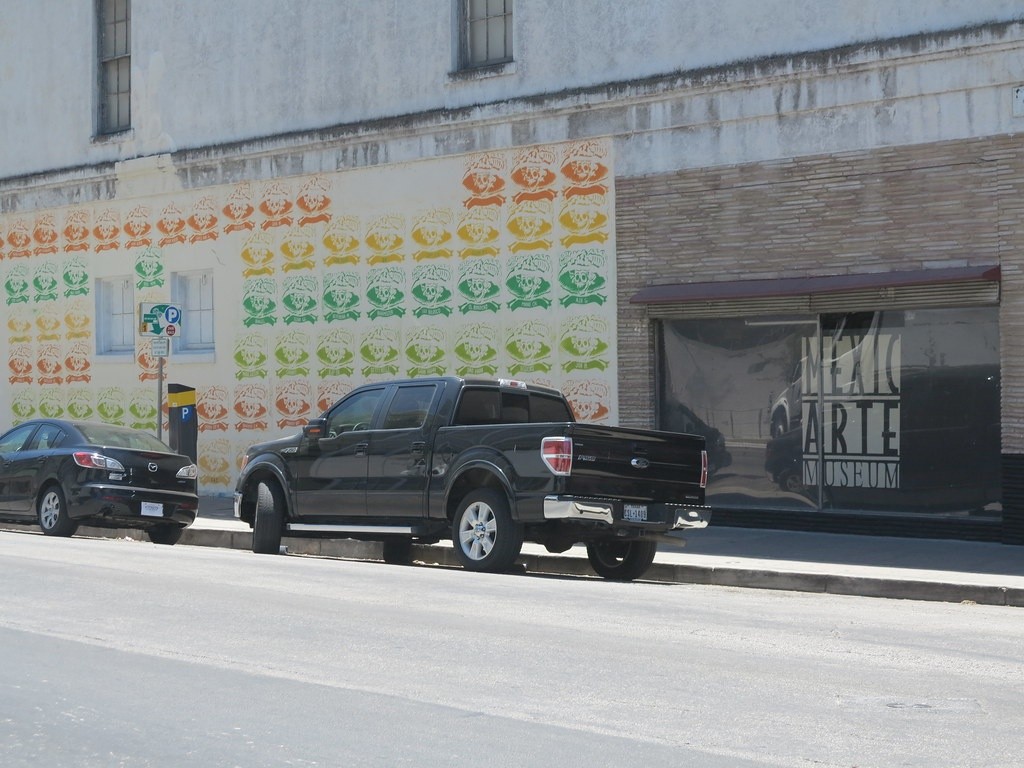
0 418 200 545
769 358 802 438
765 427 803 491
679 404 732 472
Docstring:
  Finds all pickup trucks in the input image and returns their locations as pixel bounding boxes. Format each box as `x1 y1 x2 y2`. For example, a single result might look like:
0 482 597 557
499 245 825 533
234 376 711 581
899 366 1002 509
